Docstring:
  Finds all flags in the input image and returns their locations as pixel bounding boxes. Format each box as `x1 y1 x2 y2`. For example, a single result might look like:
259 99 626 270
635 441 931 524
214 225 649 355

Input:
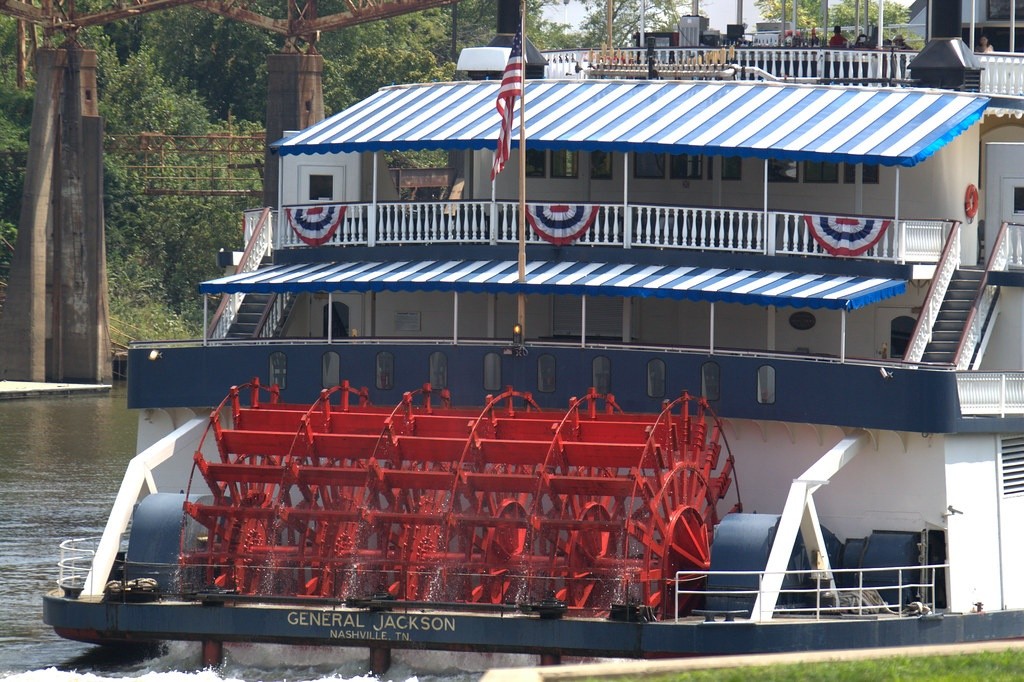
490 16 522 180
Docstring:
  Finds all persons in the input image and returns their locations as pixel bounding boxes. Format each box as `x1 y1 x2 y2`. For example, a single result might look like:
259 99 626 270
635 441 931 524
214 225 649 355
975 36 994 53
893 34 912 50
829 26 847 45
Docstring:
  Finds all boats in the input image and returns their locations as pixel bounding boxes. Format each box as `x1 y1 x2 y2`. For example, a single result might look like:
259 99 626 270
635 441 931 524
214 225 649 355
39 1 1024 673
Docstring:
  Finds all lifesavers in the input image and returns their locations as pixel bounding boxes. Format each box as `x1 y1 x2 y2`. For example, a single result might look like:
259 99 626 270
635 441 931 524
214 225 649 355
964 184 979 218
